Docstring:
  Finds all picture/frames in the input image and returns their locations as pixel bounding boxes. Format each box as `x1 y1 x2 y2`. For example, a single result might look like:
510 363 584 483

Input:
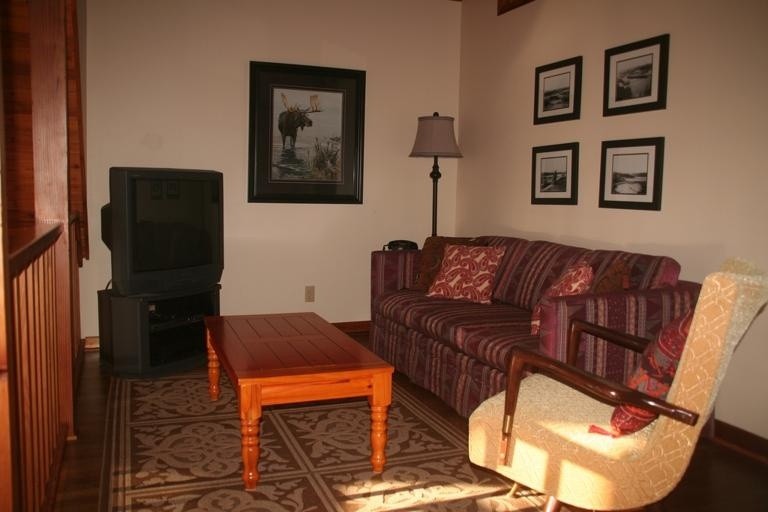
530 141 580 207
247 60 367 210
532 56 583 126
598 138 662 211
601 36 662 117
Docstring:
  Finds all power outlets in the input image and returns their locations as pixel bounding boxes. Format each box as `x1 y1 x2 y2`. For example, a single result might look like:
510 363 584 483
304 283 316 304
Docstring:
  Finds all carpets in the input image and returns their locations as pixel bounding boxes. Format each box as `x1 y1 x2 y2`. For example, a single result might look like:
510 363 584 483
97 361 560 512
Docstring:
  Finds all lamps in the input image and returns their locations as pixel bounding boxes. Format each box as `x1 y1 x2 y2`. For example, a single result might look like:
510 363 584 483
409 110 463 236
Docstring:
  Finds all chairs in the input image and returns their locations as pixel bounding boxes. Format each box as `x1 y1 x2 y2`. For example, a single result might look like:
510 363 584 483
468 258 767 512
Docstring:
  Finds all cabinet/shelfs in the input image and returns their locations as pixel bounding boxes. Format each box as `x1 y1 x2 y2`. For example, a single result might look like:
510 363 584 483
98 285 221 379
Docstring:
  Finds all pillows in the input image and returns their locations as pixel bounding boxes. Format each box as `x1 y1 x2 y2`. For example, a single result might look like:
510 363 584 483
588 302 695 437
529 260 593 334
411 236 487 293
426 242 507 306
596 253 653 292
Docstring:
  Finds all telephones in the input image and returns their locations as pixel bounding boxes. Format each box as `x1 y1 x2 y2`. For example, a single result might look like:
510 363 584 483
388 240 418 251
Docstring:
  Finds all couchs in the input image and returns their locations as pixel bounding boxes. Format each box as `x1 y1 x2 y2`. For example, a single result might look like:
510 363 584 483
371 233 680 419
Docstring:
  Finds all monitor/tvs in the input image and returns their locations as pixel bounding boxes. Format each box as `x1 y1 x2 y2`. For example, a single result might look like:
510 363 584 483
100 165 225 298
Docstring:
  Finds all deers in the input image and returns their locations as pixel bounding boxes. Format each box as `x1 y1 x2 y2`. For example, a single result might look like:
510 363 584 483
278 92 323 149
